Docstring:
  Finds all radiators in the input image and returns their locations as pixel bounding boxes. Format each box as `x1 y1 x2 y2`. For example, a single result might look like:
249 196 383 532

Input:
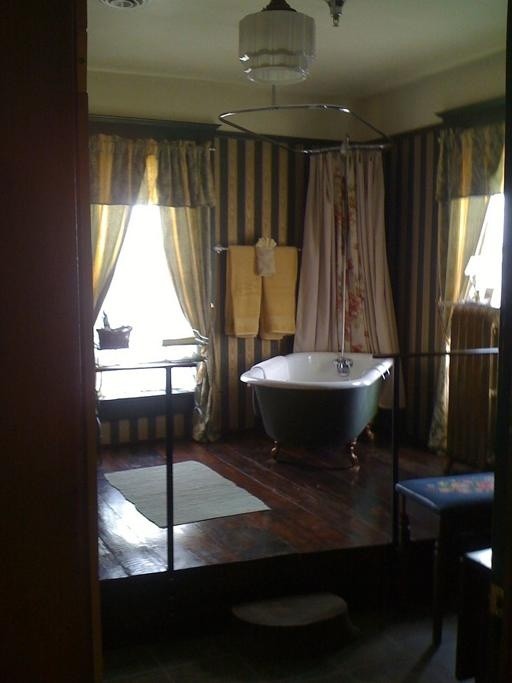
444 302 501 472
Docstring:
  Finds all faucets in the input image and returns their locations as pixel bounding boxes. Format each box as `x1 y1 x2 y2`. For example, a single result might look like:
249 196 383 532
334 356 355 374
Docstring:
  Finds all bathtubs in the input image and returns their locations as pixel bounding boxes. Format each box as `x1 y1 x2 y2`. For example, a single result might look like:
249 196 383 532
240 350 394 470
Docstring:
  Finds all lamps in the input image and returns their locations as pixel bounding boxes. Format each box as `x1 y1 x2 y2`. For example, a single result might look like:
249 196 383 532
239 0 316 86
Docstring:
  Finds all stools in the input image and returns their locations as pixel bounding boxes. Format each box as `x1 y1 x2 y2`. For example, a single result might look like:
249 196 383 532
396 472 494 646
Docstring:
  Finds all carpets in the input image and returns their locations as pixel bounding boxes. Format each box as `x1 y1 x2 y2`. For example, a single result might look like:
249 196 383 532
104 460 271 529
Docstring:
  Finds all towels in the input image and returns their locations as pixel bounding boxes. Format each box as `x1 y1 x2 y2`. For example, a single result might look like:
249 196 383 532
260 247 298 340
225 246 262 339
257 245 277 276
